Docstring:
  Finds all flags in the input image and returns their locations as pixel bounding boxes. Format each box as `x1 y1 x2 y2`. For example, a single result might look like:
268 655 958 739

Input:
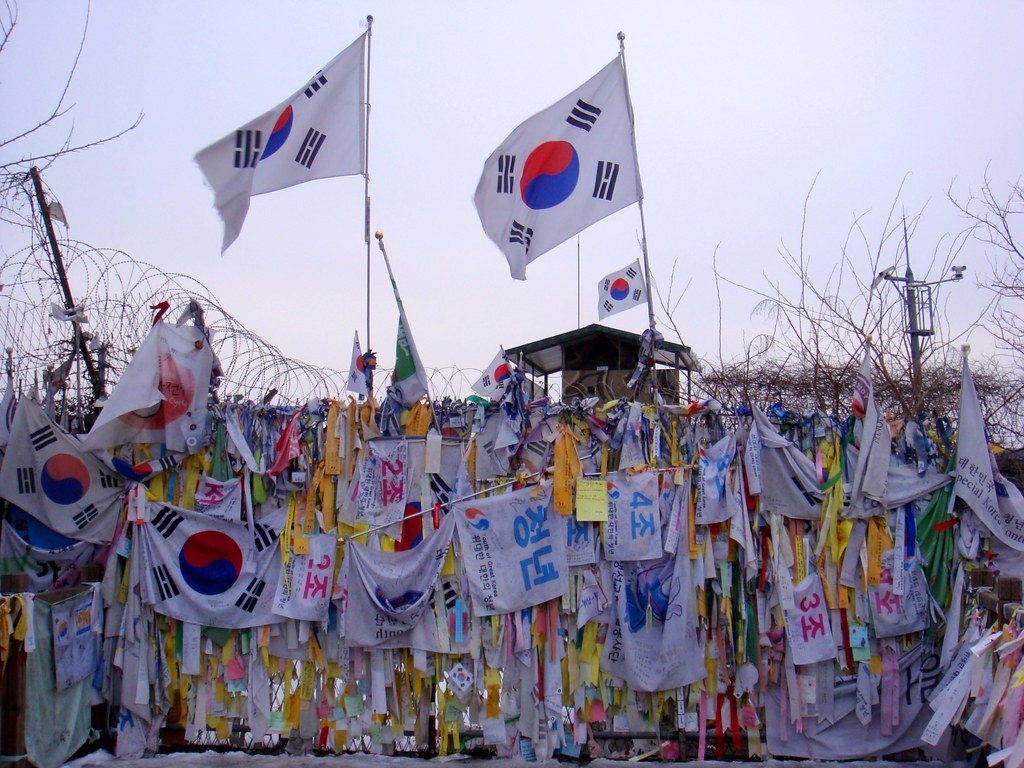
0 388 131 546
473 53 645 283
597 258 648 322
452 477 571 619
193 30 366 260
138 488 301 630
0 306 1024 693
